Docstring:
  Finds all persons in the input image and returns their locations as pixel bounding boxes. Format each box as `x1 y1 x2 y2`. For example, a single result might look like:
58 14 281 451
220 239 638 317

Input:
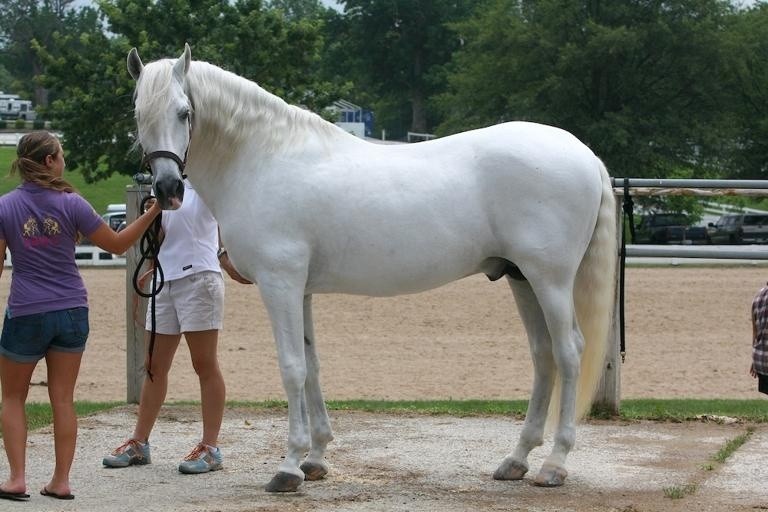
0 132 183 500
102 162 256 474
749 280 767 393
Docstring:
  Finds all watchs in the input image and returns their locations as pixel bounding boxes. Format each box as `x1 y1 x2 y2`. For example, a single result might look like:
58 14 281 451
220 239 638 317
216 247 228 257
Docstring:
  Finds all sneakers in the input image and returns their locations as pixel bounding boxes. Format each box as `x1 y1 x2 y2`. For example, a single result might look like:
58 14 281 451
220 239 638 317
102 437 152 468
178 443 223 474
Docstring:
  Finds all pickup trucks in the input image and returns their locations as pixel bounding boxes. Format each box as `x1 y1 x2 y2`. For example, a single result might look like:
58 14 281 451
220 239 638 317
635 209 709 247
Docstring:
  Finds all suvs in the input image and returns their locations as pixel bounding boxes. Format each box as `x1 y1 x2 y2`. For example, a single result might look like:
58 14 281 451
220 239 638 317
75 204 126 261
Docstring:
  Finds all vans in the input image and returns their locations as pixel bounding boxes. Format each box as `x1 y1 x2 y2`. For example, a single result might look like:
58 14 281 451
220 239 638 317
705 213 768 244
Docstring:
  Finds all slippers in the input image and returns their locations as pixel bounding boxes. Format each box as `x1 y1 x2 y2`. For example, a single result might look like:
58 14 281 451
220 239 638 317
39 483 74 501
0 481 31 500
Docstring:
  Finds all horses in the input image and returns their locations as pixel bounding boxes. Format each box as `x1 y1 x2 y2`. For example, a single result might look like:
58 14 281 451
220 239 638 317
126 41 619 494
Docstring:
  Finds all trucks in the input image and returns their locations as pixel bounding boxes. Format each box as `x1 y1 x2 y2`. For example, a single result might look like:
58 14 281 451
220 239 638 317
0 89 38 120
